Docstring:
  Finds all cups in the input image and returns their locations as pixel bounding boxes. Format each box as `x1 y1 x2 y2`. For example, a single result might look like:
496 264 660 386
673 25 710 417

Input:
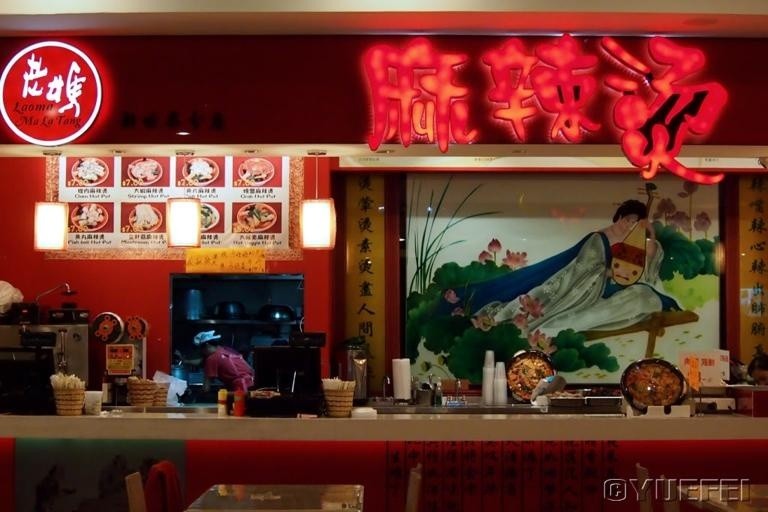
416 387 431 407
171 367 188 383
53 387 103 417
127 379 171 407
480 350 509 405
184 289 201 320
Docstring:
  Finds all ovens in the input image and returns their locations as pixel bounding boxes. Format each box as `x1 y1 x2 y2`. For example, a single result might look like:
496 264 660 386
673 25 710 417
0 322 103 415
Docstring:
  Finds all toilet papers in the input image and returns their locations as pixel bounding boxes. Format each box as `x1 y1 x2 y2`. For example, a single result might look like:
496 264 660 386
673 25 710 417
431 376 443 407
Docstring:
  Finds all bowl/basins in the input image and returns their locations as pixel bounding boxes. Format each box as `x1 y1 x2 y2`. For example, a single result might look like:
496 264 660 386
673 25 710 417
69 157 279 234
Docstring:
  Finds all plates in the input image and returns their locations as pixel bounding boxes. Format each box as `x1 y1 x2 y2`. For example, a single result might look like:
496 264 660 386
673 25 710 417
504 349 557 404
619 358 688 412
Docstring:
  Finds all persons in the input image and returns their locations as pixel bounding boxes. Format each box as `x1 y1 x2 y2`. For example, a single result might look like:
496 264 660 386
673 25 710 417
193 329 256 393
435 198 665 341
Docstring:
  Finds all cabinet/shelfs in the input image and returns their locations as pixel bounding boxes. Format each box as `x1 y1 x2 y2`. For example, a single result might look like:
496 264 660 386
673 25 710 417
164 269 307 394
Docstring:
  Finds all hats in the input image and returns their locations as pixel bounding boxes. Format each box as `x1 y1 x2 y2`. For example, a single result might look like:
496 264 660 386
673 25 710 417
192 330 221 347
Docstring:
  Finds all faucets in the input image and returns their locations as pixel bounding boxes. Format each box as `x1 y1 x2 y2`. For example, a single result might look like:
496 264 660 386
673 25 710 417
382 375 390 398
455 378 461 401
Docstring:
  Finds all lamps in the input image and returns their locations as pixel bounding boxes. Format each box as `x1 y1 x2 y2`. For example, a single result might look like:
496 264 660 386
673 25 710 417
31 150 70 253
298 147 340 250
167 151 202 250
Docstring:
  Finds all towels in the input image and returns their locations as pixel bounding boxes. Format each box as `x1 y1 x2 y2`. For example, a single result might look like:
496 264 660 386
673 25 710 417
392 358 411 400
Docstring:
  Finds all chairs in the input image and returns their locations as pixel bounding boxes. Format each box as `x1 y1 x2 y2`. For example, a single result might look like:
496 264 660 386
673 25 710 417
630 463 767 512
126 457 421 511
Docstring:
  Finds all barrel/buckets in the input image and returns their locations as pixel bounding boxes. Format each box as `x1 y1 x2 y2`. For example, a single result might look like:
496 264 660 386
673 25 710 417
171 364 188 382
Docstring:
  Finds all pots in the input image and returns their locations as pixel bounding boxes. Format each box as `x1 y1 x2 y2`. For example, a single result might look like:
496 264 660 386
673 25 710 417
209 300 298 322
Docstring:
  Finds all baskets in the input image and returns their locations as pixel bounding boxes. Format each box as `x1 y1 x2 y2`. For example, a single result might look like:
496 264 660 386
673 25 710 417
153 382 170 406
53 388 86 416
127 380 156 407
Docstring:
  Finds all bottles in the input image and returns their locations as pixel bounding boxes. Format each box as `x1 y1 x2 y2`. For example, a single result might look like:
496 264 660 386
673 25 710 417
434 376 464 407
216 388 248 417
